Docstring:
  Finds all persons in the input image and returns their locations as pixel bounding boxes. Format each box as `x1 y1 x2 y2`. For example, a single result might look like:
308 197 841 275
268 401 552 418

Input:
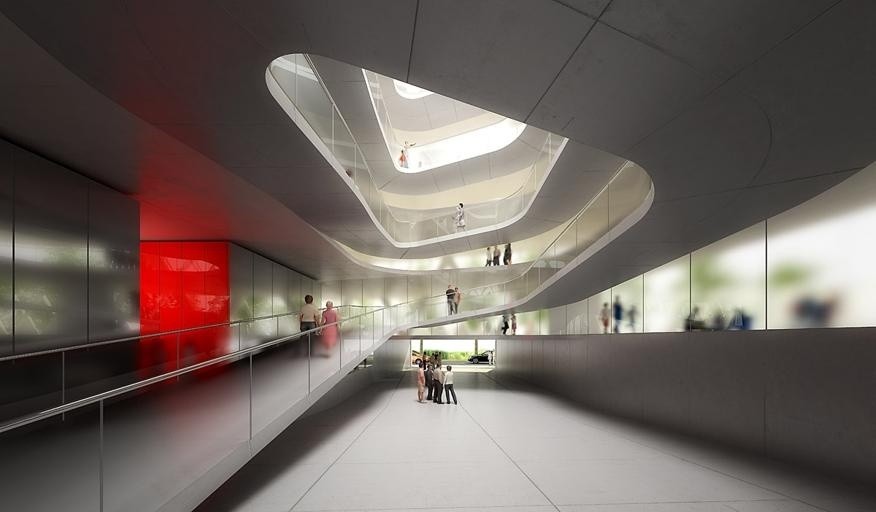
613 295 622 333
300 295 322 356
454 288 460 314
321 301 340 357
455 203 466 232
599 302 611 333
628 306 639 332
725 308 744 330
446 285 455 315
501 316 509 335
399 149 408 168
417 351 457 405
485 247 492 266
493 245 501 266
687 307 705 327
503 245 509 265
508 243 512 264
511 313 517 335
